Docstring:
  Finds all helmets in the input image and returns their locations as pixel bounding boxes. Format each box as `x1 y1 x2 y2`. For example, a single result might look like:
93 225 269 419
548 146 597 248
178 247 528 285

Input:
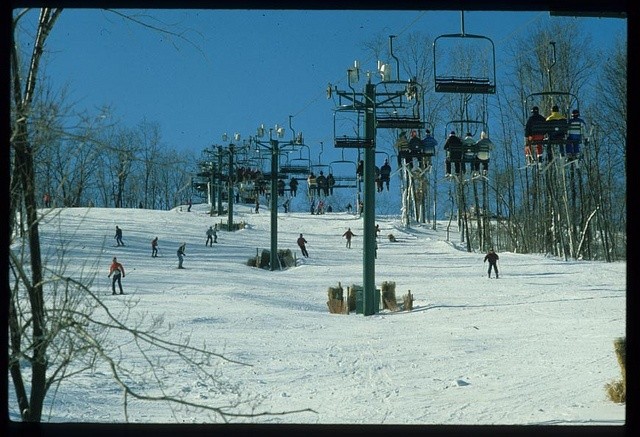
531 106 538 113
571 109 580 117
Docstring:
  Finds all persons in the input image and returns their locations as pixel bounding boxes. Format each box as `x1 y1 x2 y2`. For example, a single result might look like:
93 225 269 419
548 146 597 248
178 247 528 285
289 177 298 198
44 192 49 207
375 165 380 193
375 224 381 240
283 199 289 213
316 201 322 214
380 159 391 193
394 132 414 171
525 106 546 172
442 131 462 179
565 109 589 163
254 198 259 214
186 199 191 212
114 225 124 246
483 247 499 280
462 132 477 180
408 130 422 171
307 172 316 196
213 224 219 243
421 129 438 173
356 160 364 192
297 234 309 257
151 237 158 258
327 173 334 195
474 130 494 178
545 105 568 164
176 242 186 269
310 200 315 214
107 257 125 294
342 227 357 248
205 226 214 247
316 170 327 198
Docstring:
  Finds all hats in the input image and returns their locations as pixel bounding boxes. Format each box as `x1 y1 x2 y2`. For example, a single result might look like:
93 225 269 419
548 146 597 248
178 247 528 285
481 131 488 139
384 158 388 161
426 129 430 133
399 132 406 137
450 131 455 134
